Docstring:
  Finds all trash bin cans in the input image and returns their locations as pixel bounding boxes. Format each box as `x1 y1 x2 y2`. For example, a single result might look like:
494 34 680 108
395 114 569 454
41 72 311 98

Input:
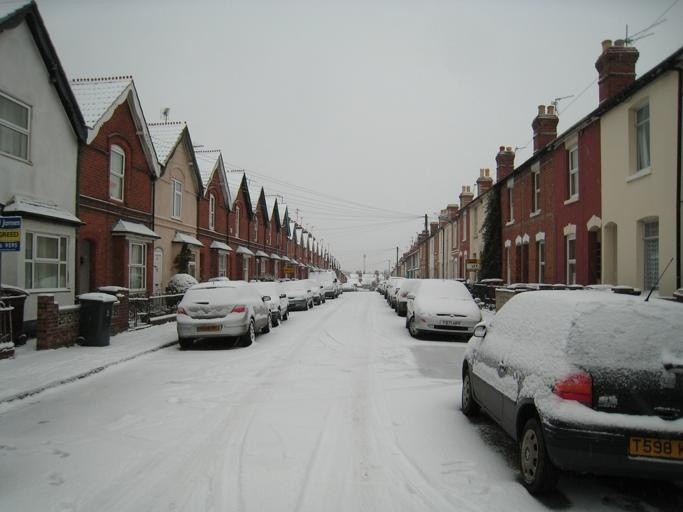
75 293 117 347
0 288 27 346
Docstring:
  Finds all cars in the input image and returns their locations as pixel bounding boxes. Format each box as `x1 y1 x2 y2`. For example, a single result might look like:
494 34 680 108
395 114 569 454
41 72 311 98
174 279 359 349
456 255 682 509
373 275 483 341
